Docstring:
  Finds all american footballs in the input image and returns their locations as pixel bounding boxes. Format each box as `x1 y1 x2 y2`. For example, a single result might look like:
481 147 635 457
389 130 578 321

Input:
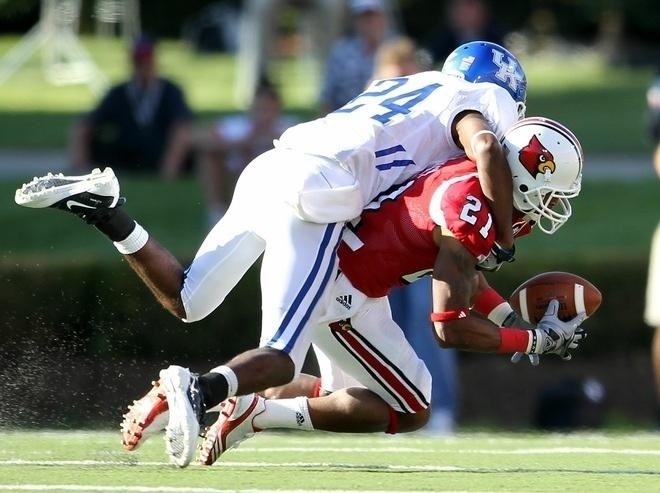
508 271 603 326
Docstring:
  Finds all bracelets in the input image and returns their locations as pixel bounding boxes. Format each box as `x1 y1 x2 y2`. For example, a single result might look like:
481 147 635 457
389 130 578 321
496 327 529 355
471 287 506 316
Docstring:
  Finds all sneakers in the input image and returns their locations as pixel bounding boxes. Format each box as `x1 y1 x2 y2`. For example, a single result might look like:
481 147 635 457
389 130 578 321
15 167 126 224
119 365 268 467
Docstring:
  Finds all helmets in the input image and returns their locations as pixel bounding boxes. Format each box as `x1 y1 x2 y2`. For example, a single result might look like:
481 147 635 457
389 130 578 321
442 40 527 119
499 117 584 210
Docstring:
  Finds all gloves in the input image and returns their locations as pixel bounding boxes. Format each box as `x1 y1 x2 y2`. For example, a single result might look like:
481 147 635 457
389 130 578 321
503 300 590 366
477 240 515 272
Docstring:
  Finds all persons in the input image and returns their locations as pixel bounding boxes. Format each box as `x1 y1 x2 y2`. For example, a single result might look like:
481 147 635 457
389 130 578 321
163 73 301 227
82 37 197 180
118 117 589 467
640 86 660 402
14 41 527 469
362 35 463 433
321 2 432 109
